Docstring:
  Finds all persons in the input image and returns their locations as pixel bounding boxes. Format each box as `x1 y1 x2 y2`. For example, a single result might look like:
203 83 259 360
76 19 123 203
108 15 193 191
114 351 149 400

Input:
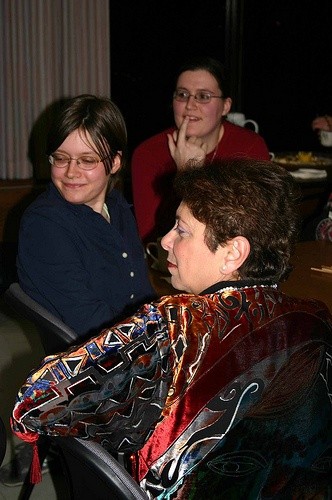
16 94 158 339
131 56 270 244
10 157 299 500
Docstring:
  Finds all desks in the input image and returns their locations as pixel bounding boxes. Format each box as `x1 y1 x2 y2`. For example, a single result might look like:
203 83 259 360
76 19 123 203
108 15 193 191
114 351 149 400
150 241 332 313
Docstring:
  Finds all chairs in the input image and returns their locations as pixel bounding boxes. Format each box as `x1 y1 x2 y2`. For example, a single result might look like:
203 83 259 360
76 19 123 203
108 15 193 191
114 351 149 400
10 282 82 356
46 436 150 500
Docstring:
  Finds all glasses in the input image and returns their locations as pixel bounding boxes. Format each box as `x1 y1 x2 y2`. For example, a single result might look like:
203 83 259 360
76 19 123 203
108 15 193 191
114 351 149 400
173 88 225 104
49 151 115 171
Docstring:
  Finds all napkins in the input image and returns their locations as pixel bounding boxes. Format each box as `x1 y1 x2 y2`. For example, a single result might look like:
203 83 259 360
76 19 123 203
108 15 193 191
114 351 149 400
291 168 328 180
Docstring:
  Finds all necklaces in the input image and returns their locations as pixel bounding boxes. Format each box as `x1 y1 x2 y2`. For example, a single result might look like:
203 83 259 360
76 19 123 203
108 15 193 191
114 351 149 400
215 284 277 294
